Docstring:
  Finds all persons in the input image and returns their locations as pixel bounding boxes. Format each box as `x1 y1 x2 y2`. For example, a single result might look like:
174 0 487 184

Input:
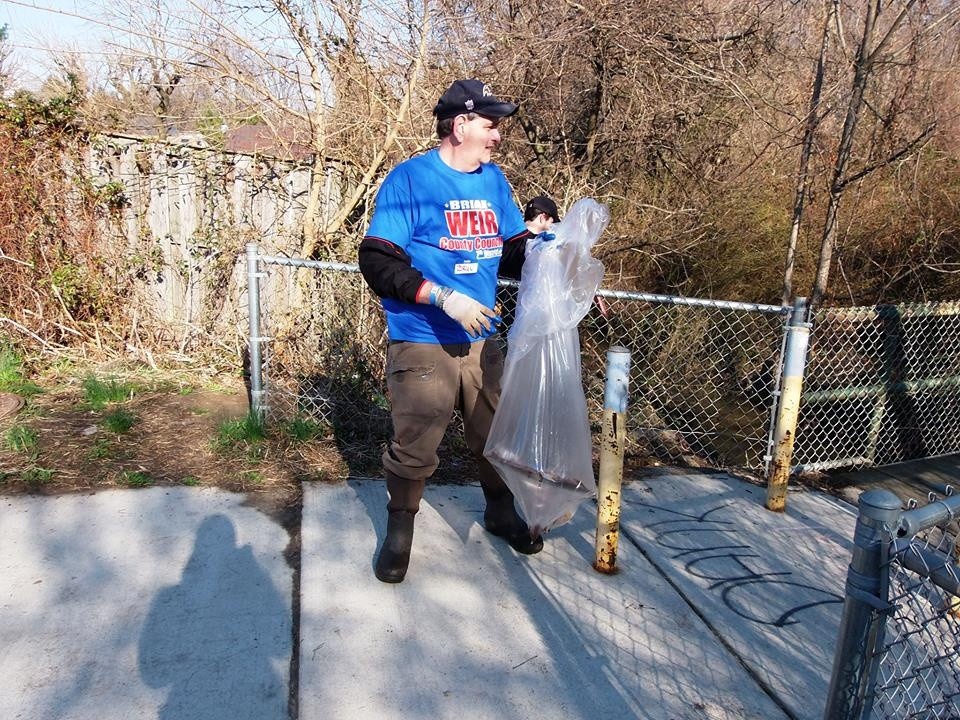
498 196 606 351
359 79 555 582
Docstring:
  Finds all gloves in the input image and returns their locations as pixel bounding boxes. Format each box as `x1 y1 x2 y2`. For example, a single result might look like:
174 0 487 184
533 233 557 252
442 290 502 340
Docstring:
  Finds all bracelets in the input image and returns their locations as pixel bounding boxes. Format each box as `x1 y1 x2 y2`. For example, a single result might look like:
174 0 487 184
430 286 454 309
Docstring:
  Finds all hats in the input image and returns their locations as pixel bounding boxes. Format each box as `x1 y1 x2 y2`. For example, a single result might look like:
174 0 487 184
435 79 521 120
527 197 562 223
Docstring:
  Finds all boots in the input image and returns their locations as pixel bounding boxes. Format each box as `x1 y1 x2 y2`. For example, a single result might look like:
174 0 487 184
374 471 425 583
476 459 544 554
